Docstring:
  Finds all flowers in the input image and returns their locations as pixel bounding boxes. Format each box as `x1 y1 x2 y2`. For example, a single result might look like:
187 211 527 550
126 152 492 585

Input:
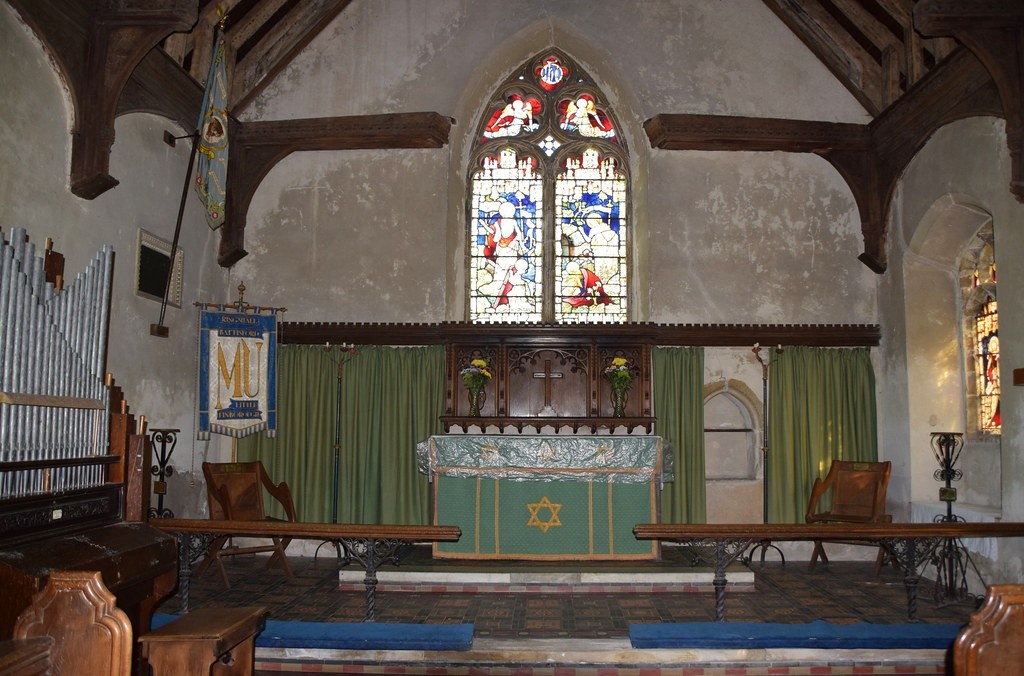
458 357 493 415
604 358 640 413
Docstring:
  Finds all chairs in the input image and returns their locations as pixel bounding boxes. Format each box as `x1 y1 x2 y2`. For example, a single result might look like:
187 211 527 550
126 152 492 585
805 460 899 583
194 460 298 588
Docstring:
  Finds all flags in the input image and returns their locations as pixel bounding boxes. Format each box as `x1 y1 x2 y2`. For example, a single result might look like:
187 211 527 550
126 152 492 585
196 36 233 232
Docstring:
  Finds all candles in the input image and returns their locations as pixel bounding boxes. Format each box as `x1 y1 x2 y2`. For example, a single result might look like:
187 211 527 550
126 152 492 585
343 343 346 347
778 345 781 349
756 343 759 348
326 342 329 347
351 344 354 348
754 344 757 349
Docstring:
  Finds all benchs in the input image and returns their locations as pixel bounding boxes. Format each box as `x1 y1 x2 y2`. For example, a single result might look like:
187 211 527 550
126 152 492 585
138 607 268 676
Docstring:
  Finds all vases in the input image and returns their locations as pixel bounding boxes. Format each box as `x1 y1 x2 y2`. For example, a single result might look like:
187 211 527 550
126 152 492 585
467 380 483 417
606 374 634 418
